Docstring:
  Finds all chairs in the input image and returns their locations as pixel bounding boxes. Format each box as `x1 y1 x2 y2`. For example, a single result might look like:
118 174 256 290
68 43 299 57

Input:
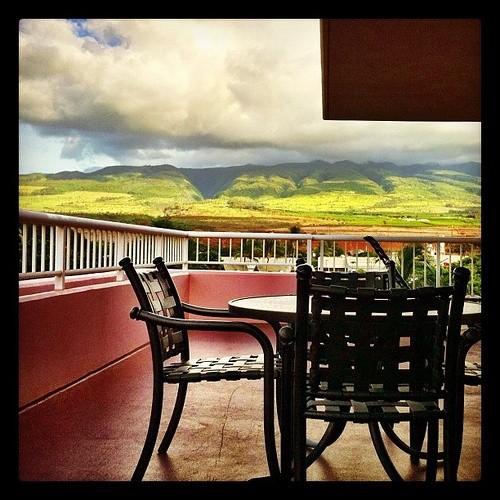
290 265 472 482
118 256 283 482
311 260 396 371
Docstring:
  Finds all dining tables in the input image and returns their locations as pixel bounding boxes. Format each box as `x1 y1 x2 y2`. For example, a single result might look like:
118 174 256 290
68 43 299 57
229 292 481 481
